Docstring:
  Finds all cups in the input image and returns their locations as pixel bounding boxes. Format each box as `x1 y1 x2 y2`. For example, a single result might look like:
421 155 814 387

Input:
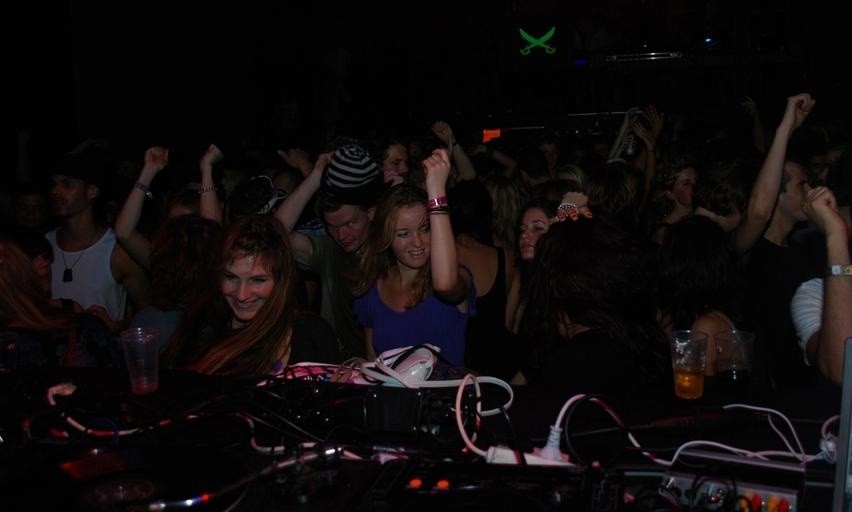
714 324 761 403
119 326 165 397
669 326 708 398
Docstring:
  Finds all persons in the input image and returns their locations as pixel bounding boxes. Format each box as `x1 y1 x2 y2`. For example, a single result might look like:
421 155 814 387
1 93 851 410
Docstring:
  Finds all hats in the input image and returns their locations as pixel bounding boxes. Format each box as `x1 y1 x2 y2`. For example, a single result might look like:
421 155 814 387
318 143 386 209
